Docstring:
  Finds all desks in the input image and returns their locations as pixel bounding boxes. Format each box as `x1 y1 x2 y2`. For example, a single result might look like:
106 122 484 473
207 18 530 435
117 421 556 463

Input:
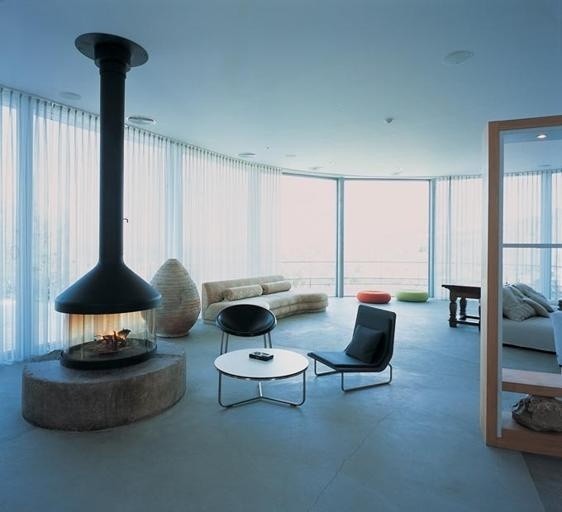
441 284 480 332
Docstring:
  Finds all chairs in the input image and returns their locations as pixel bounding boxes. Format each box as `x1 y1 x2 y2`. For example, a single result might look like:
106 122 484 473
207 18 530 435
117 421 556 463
306 304 397 393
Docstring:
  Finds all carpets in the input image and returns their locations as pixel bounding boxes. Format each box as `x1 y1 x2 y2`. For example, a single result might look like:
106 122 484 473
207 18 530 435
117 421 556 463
521 450 562 512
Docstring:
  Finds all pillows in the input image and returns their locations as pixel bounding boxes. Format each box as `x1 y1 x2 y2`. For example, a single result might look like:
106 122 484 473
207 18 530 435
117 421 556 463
225 280 292 301
502 283 555 322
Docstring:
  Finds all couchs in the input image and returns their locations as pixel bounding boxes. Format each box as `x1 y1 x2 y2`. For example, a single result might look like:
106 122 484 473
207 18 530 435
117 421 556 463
202 275 329 325
502 283 556 355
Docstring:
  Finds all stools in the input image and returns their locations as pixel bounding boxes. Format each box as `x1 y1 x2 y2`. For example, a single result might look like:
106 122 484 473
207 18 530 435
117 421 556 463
215 304 277 356
